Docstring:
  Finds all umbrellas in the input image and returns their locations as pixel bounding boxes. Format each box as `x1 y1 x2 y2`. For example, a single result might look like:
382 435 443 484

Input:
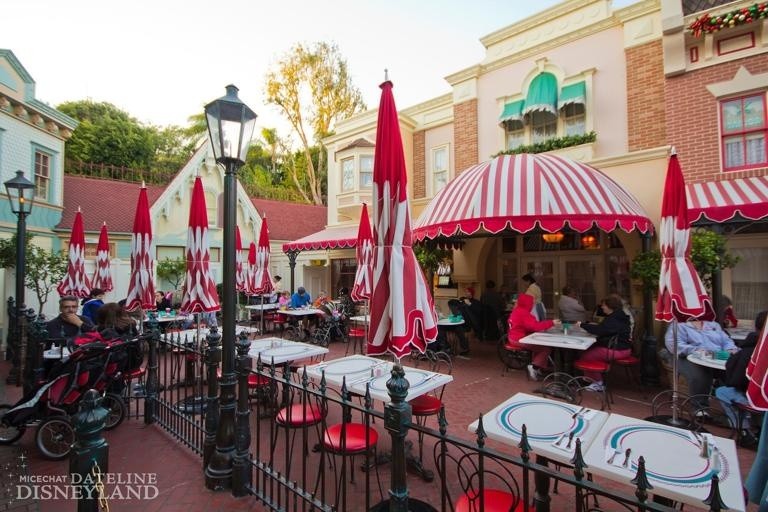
177 174 222 402
251 216 274 340
93 222 114 295
236 224 245 325
365 82 438 366
745 313 768 412
124 188 161 386
59 211 90 299
644 153 716 420
245 241 255 323
350 205 374 345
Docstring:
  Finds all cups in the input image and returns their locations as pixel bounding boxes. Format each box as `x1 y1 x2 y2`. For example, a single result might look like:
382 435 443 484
165 307 171 314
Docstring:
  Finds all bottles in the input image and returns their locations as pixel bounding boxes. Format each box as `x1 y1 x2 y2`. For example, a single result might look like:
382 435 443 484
697 435 709 458
51 343 56 355
709 446 721 471
563 327 567 336
701 349 706 360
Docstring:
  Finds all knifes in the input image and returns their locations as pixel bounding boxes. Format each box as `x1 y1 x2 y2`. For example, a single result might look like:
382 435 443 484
622 448 632 467
566 431 574 448
572 407 584 419
691 431 718 449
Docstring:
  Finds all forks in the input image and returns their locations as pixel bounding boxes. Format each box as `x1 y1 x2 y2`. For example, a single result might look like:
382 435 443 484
556 433 570 445
607 448 624 464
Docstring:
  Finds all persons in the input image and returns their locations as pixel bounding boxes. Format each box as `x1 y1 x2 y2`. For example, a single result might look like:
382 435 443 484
272 275 347 334
46 295 95 338
448 273 639 393
665 320 742 426
156 291 171 311
95 304 144 371
81 289 106 324
715 311 767 449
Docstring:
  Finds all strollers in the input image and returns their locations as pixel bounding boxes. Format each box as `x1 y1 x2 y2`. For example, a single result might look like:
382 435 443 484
0 329 155 461
312 298 350 347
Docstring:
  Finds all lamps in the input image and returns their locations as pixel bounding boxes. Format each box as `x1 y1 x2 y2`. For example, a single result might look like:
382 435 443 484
542 231 564 244
579 230 598 250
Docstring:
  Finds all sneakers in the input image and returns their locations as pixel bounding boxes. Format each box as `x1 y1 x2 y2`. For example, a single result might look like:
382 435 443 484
582 381 607 392
527 364 539 381
537 367 544 376
459 348 470 356
694 408 710 417
739 427 759 447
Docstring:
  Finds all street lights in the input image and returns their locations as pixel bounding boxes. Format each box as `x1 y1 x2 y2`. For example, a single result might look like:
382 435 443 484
4 168 39 383
197 83 262 491
